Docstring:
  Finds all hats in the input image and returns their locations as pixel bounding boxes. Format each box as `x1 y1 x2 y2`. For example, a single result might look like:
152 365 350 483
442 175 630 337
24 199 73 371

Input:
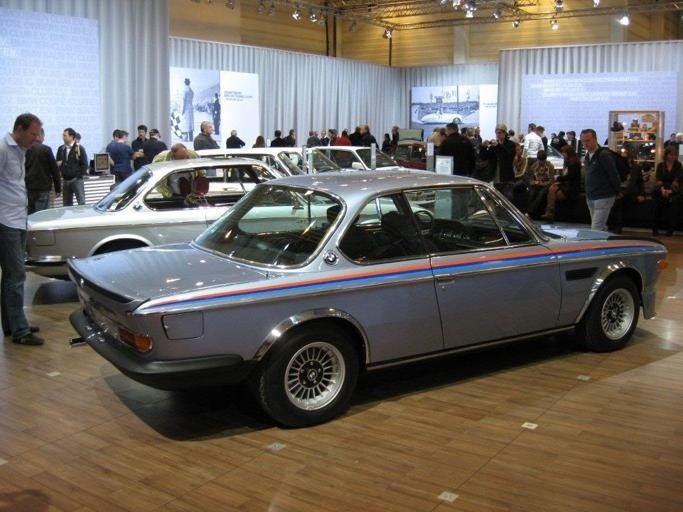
495 122 508 135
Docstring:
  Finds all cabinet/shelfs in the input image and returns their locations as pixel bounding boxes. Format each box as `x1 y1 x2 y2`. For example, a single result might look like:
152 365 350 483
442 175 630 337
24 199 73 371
607 110 664 180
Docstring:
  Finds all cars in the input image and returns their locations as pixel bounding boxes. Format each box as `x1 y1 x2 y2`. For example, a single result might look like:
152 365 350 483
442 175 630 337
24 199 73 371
421 108 463 124
64 170 669 430
518 143 565 170
24 158 434 282
143 146 406 200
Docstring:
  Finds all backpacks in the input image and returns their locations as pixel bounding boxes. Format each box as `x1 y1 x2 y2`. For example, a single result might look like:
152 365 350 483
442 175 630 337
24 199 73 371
609 149 629 182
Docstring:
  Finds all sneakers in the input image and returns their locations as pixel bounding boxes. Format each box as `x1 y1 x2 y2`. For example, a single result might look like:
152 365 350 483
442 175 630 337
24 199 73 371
12 327 44 345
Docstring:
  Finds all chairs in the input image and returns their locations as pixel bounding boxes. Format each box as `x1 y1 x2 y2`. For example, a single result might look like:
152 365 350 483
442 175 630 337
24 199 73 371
374 212 436 256
321 206 369 252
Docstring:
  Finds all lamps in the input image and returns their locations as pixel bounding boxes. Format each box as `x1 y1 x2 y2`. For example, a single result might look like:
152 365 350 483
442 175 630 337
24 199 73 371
439 0 563 32
187 0 399 39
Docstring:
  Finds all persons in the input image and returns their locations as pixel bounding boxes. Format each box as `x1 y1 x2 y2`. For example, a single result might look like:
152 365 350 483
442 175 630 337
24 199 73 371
193 123 399 179
106 125 206 198
0 110 89 345
427 119 683 233
181 78 194 141
212 92 220 135
